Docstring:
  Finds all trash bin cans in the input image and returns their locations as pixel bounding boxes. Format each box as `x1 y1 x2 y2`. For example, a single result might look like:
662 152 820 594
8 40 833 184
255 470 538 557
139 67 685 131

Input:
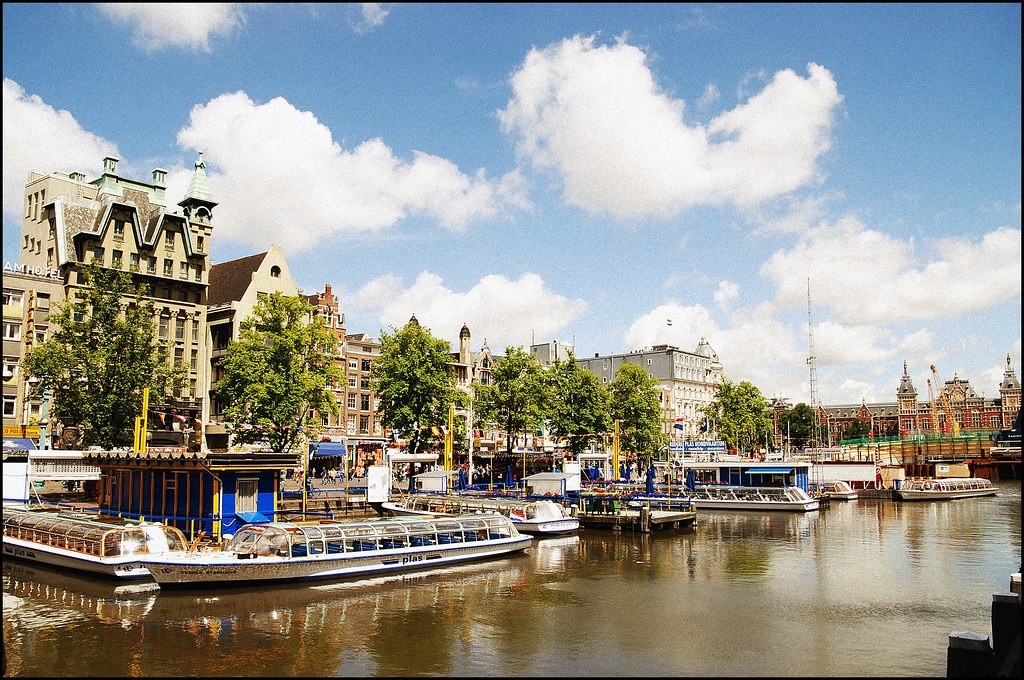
604 495 615 516
593 496 603 515
568 493 580 511
613 500 622 515
585 493 594 515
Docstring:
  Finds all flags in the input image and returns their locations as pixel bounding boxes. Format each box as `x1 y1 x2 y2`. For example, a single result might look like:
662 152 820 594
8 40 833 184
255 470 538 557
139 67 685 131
673 417 683 430
697 417 707 433
466 427 618 450
157 412 448 436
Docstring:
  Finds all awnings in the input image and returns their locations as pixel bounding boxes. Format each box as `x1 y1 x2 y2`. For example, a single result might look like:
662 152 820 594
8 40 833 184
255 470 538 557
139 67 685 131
3 437 37 457
309 442 346 459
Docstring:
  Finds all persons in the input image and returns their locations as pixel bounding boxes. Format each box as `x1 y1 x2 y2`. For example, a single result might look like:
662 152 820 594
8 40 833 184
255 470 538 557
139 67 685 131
405 464 410 477
298 471 310 495
321 462 352 485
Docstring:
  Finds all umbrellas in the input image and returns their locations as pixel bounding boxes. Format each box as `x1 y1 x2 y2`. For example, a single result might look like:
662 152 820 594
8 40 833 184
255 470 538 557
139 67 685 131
646 463 656 497
687 468 695 508
625 463 630 483
459 469 466 490
506 466 512 487
621 463 625 479
595 463 599 479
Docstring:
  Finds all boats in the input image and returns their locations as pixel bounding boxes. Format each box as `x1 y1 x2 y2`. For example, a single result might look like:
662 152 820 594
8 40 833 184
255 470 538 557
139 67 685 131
810 480 859 500
3 508 171 581
633 485 820 513
138 509 535 591
381 492 579 537
895 477 1000 501
